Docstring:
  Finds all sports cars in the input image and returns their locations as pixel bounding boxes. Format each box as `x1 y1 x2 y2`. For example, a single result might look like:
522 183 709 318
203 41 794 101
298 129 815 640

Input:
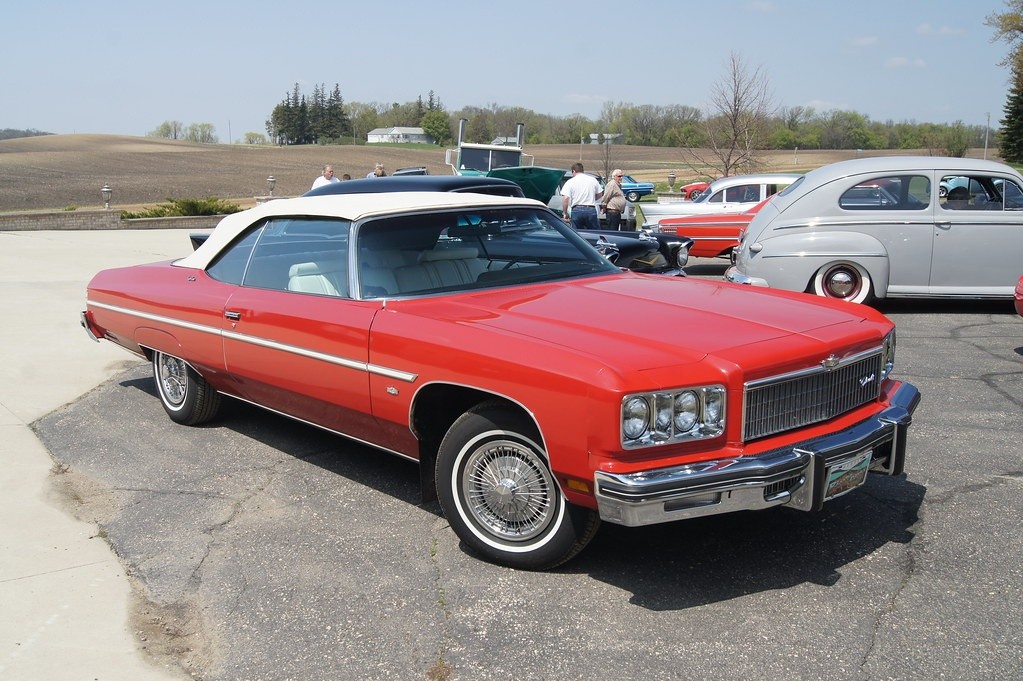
80 193 923 573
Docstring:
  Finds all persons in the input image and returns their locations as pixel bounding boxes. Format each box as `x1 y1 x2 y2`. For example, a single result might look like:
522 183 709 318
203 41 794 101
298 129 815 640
367 162 384 179
342 174 351 181
560 163 604 230
598 168 627 231
376 169 385 177
942 186 973 210
311 164 341 191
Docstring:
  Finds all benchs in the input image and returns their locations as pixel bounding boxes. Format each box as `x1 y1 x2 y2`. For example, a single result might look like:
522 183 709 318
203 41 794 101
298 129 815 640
226 246 428 292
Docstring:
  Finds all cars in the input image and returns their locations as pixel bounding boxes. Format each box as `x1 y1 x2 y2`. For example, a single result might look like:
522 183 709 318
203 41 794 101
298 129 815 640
722 155 1023 309
680 172 771 202
619 175 655 202
189 173 697 281
638 172 803 235
937 172 1005 200
658 179 925 269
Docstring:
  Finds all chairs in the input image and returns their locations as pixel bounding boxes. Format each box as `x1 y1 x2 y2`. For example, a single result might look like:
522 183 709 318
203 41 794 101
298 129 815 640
287 260 351 297
418 246 487 288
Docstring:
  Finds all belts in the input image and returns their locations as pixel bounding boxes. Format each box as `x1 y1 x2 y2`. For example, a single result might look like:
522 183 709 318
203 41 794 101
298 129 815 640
571 205 595 208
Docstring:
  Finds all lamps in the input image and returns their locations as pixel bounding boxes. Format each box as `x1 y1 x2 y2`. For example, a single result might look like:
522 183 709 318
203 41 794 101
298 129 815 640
668 172 676 192
266 176 277 196
100 182 113 209
